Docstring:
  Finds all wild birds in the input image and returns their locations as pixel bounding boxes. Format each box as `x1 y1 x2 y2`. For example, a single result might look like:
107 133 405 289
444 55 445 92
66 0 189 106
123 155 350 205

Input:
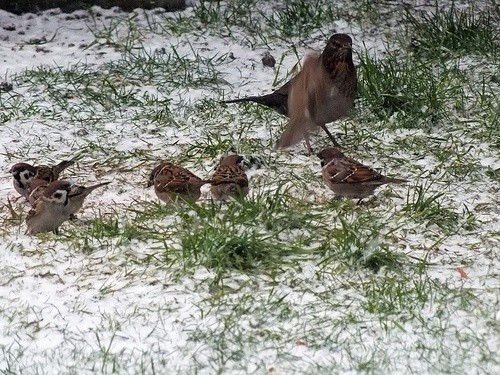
313 147 411 206
4 149 112 234
146 154 249 209
219 33 360 156
